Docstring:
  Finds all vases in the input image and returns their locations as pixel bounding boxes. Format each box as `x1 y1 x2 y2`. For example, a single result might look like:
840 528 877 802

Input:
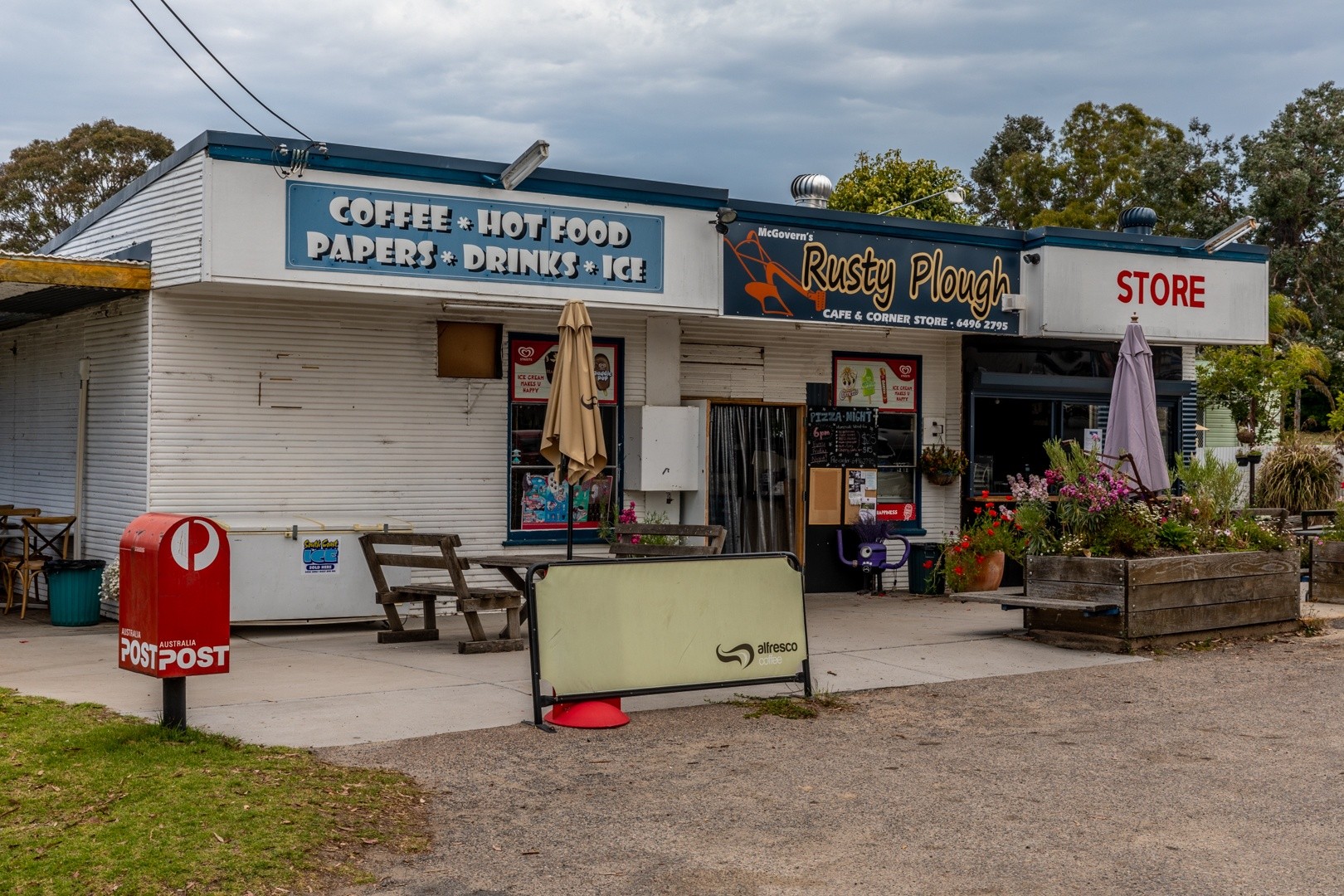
1309 539 1344 604
925 473 956 485
1024 549 1300 638
954 550 1004 593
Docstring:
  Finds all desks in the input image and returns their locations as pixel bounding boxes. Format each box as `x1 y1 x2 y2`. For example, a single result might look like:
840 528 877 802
0 529 74 608
469 554 610 639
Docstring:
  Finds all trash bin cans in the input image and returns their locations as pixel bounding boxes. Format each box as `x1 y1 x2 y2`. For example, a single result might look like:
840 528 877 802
41 559 104 626
910 541 945 595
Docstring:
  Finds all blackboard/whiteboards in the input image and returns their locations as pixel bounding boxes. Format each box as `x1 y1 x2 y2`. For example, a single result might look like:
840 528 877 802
805 403 878 468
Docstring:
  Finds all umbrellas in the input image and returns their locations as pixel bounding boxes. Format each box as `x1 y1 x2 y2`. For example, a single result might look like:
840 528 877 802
540 300 607 560
1103 311 1170 492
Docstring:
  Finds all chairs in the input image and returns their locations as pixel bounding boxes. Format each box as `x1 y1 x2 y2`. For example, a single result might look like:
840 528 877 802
0 504 76 619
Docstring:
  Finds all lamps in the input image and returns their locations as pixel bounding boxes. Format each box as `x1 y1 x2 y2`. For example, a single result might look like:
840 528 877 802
501 140 549 191
1022 254 1040 264
877 187 966 216
1203 216 1259 254
716 207 737 235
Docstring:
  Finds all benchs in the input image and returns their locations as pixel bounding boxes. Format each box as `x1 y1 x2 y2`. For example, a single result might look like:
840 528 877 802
949 593 1117 612
609 523 727 558
358 533 524 654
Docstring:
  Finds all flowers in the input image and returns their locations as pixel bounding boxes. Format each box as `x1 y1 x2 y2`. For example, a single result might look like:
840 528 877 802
920 443 968 475
98 556 119 601
923 491 1026 593
1007 436 1304 559
596 496 679 559
1315 508 1344 552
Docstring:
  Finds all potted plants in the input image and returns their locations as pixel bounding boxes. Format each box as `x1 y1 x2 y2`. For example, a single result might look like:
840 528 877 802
1236 428 1256 443
1248 450 1262 464
1236 448 1249 466
